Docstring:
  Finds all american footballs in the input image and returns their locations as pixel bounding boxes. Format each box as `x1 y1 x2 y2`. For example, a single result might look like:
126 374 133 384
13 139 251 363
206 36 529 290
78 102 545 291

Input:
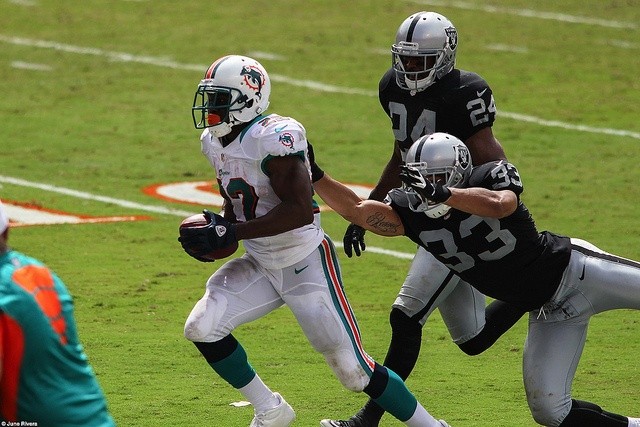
180 214 239 259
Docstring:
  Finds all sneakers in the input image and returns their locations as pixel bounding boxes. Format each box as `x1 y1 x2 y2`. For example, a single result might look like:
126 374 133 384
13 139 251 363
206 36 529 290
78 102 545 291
321 418 378 427
250 392 295 427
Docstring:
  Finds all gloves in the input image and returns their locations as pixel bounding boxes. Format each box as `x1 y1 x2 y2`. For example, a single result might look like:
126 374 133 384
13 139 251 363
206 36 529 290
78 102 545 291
343 223 366 257
399 165 453 204
181 209 232 248
307 141 324 182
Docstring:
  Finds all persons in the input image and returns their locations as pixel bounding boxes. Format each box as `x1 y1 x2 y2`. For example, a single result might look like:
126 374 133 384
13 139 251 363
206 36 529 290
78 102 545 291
178 55 450 427
0 201 119 427
317 11 528 427
308 133 640 426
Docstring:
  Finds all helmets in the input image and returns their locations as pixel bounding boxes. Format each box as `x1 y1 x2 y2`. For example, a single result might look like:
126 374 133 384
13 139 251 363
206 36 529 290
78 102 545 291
392 11 459 91
404 133 472 220
192 55 270 138
